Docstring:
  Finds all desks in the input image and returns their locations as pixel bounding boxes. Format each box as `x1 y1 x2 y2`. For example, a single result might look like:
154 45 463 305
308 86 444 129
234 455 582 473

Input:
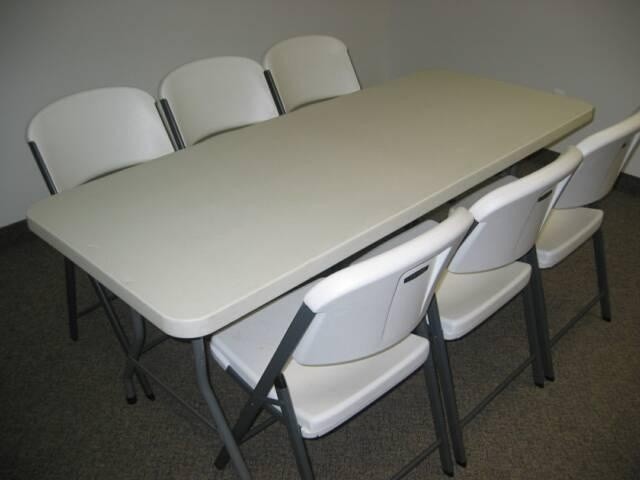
26 67 596 479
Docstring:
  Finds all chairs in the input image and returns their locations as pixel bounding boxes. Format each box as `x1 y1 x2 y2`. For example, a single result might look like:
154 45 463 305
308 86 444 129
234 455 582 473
338 147 584 466
209 205 477 478
155 54 281 148
23 84 184 345
264 32 361 115
445 106 640 381
